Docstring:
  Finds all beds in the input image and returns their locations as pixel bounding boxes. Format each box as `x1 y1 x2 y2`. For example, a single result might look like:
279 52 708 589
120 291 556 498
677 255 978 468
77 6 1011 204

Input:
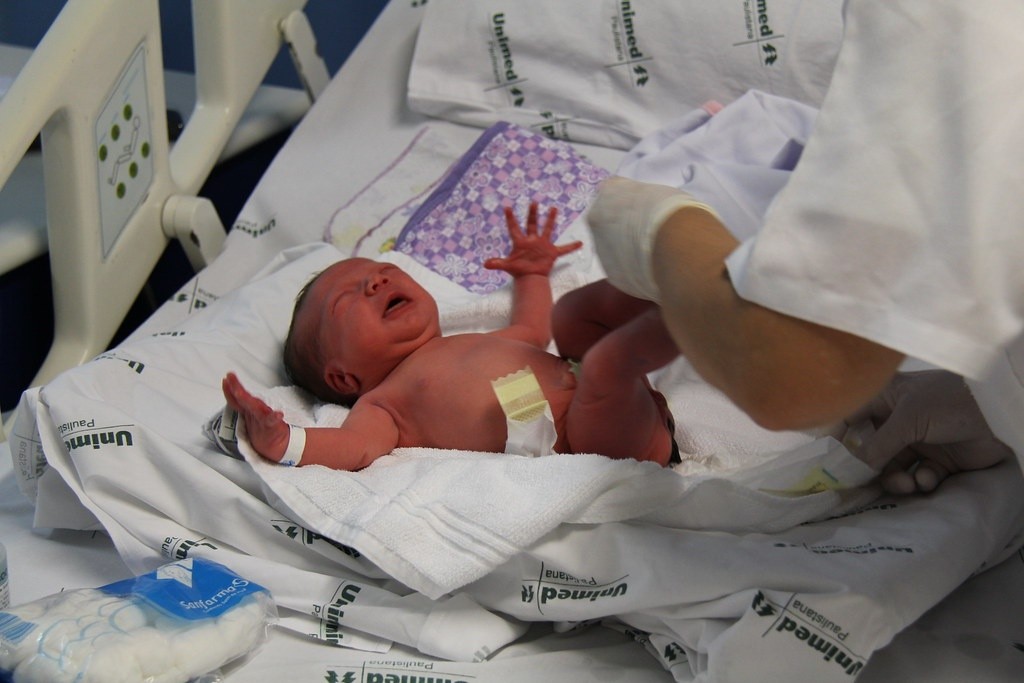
0 0 1022 683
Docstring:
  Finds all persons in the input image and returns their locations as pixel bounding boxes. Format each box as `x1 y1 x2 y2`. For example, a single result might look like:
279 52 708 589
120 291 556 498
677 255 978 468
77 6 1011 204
222 202 683 471
586 0 1023 493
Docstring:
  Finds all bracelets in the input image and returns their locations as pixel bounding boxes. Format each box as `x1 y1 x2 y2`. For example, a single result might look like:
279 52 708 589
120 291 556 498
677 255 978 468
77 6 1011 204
278 423 306 467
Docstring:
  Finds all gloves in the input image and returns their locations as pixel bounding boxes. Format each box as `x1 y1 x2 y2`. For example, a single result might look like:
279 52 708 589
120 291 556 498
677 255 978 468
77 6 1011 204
588 172 728 304
